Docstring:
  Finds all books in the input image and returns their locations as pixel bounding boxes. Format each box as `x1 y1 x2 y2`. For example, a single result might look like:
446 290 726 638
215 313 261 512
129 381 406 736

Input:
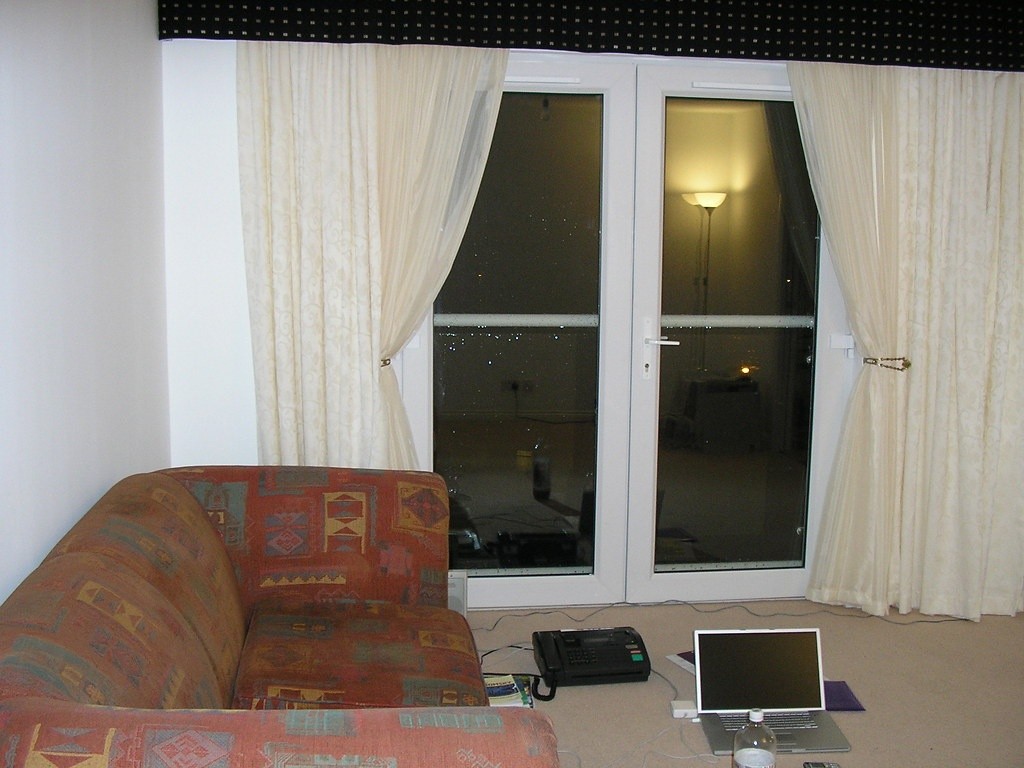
484 674 535 709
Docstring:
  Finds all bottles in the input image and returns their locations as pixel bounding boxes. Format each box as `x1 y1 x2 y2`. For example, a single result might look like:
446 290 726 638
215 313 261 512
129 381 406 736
530 439 551 499
732 708 777 768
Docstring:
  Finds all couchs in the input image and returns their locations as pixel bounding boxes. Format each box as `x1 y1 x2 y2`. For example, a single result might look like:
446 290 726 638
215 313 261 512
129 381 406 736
0 464 559 768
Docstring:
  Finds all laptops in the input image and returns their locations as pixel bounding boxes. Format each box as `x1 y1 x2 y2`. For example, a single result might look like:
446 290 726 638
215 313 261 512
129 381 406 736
693 628 851 757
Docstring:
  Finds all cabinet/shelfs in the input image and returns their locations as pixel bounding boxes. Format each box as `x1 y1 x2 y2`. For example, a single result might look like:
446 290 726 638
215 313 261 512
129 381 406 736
666 377 772 455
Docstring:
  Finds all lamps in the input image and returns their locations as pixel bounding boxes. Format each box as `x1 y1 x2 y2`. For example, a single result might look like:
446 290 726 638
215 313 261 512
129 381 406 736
678 192 728 372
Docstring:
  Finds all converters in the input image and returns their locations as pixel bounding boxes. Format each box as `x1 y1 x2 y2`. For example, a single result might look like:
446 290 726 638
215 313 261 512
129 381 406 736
670 701 698 718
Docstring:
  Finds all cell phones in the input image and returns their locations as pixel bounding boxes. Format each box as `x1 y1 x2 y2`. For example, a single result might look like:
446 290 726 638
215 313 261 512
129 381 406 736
803 761 841 768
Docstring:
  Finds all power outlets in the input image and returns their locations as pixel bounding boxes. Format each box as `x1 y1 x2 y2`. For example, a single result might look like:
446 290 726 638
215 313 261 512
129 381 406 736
501 379 535 392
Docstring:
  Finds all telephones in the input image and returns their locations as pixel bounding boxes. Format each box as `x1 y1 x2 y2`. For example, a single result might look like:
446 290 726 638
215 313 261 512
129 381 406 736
531 625 651 687
498 529 578 568
